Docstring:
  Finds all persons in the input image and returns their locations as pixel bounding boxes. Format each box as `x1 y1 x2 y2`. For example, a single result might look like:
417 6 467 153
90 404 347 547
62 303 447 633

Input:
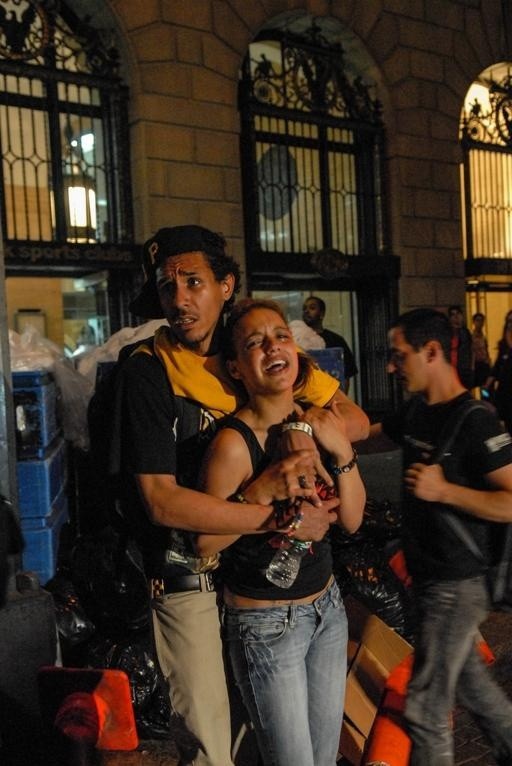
302 297 359 394
353 306 512 764
109 226 370 764
194 300 367 765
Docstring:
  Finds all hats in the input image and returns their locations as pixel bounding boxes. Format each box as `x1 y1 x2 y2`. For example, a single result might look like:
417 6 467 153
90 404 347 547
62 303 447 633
143 224 226 273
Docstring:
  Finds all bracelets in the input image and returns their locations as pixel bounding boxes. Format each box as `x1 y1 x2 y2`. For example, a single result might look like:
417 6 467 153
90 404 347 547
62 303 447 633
333 449 358 474
281 422 312 436
234 493 248 504
276 499 304 531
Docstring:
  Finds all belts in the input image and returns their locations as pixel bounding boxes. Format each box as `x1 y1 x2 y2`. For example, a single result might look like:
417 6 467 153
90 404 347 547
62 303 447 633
165 574 219 592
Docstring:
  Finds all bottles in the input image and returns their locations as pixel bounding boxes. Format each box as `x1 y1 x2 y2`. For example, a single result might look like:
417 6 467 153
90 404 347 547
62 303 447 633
265 491 327 591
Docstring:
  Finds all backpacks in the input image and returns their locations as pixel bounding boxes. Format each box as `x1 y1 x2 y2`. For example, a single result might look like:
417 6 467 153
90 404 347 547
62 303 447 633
406 396 512 610
87 341 175 594
